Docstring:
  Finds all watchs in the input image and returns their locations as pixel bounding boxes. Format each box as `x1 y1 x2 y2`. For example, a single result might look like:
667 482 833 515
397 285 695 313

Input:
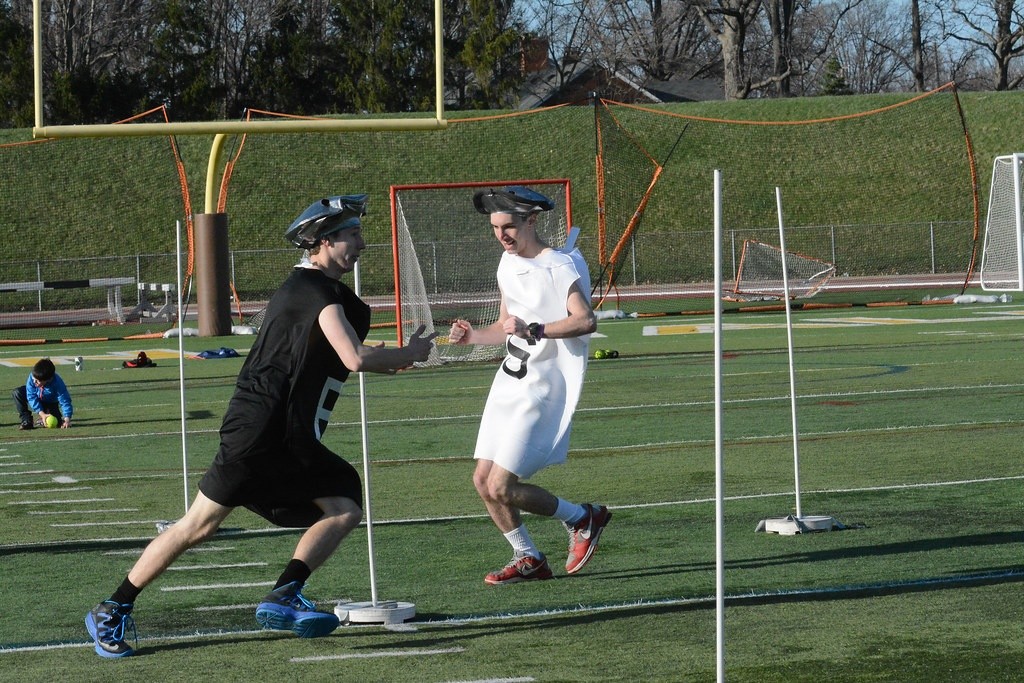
528 323 538 335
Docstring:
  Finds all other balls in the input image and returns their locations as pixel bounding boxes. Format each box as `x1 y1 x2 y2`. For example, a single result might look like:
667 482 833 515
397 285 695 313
46 415 58 428
594 349 612 359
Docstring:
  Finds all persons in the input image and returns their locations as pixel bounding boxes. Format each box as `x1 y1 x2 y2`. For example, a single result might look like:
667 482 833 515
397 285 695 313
12 358 74 431
84 193 440 658
448 187 613 584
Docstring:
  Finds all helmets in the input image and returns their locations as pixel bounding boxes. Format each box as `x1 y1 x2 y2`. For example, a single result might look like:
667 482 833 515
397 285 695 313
286 193 370 249
472 187 555 217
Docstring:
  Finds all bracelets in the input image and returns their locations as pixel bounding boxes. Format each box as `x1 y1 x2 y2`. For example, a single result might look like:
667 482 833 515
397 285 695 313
537 324 544 342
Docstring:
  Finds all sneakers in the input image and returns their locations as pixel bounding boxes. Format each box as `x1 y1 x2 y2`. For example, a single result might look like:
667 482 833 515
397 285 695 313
83 598 140 659
484 552 552 586
565 503 612 573
256 589 339 638
18 420 33 430
